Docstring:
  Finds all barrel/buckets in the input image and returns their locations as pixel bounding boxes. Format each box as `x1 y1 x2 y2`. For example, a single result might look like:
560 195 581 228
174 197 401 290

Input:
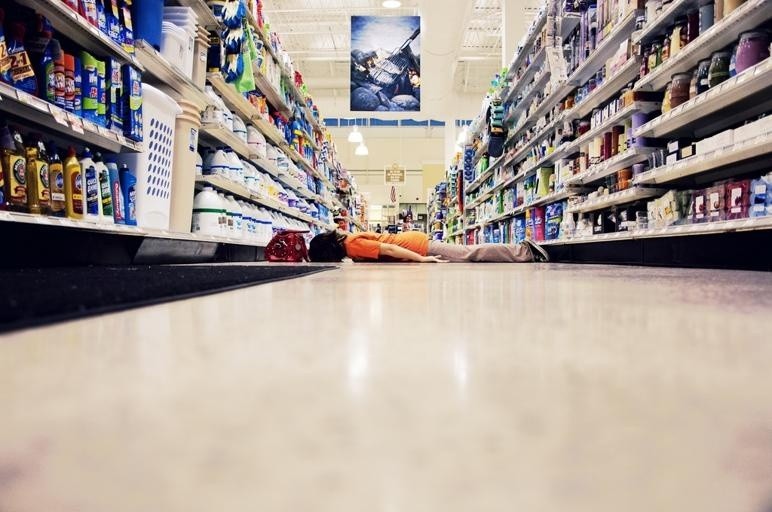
191 83 319 250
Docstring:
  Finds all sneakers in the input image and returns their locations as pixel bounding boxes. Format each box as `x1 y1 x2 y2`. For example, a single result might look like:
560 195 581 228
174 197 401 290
526 240 550 263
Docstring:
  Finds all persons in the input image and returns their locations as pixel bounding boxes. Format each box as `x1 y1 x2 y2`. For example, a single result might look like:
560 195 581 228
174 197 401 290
307 227 551 263
401 216 411 232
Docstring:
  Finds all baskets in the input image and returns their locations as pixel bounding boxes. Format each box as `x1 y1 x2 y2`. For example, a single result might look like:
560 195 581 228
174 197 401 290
115 83 182 233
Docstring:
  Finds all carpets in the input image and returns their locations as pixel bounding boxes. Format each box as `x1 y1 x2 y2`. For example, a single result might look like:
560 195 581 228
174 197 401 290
0 264 340 335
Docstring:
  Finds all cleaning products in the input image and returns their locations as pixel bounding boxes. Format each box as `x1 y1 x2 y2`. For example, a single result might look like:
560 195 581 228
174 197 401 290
0 0 145 228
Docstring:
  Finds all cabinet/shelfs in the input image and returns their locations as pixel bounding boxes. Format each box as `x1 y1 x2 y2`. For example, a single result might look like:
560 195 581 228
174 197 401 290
428 0 772 272
0 1 369 246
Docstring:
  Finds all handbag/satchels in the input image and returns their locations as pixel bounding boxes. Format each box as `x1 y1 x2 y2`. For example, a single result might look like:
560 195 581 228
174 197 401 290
264 231 309 261
